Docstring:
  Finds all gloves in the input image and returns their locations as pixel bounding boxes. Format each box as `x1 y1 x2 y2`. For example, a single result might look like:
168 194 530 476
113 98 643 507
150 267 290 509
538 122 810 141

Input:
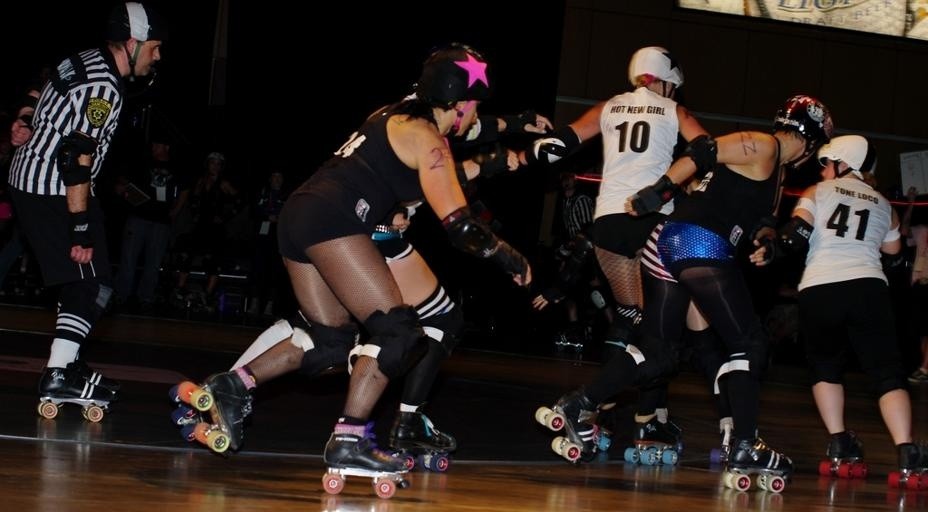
18 112 33 138
494 238 527 283
755 227 776 265
500 111 537 134
471 147 512 179
65 209 97 251
632 175 674 215
540 284 568 304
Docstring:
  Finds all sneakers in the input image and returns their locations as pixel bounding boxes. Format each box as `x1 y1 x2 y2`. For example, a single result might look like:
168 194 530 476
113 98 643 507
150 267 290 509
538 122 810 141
264 300 273 316
247 298 258 314
908 369 928 382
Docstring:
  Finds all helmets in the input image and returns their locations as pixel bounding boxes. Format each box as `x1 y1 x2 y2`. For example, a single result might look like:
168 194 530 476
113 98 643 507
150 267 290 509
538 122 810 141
99 4 171 46
417 42 489 103
773 94 833 146
817 135 878 181
207 152 224 163
629 45 685 88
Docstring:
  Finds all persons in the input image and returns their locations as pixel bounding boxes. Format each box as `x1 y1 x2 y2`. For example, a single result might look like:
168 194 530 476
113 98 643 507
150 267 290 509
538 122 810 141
2 0 163 401
900 185 928 383
654 297 734 455
248 168 290 315
177 148 252 313
624 93 836 471
791 135 927 471
520 44 714 466
206 112 555 451
206 41 533 472
560 173 624 343
115 137 189 305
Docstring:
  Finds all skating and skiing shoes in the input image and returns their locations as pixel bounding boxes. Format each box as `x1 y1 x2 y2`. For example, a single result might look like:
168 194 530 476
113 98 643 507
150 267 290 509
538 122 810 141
536 386 612 461
194 291 213 313
38 365 111 422
818 431 867 476
552 334 584 352
73 360 121 400
710 417 734 463
887 442 927 491
168 363 253 453
724 437 796 493
170 286 195 311
322 418 409 500
625 411 688 466
391 411 460 472
